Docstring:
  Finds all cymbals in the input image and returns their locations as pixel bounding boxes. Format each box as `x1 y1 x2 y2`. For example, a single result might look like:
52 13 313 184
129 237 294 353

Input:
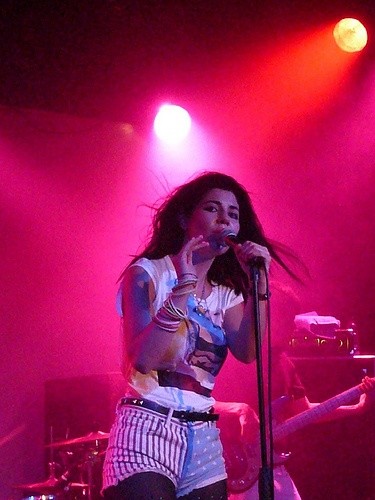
15 477 89 495
42 430 111 450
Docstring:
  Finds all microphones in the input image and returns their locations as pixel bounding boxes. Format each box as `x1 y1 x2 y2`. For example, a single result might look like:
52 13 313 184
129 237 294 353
217 229 260 264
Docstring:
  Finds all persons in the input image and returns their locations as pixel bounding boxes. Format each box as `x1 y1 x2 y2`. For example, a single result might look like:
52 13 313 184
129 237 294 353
210 279 375 500
100 173 271 500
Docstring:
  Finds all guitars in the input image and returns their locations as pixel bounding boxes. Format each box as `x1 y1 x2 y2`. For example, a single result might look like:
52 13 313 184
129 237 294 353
223 376 375 494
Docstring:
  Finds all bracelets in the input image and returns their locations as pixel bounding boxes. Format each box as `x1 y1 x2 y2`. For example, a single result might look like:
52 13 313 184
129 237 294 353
249 286 271 301
152 272 200 333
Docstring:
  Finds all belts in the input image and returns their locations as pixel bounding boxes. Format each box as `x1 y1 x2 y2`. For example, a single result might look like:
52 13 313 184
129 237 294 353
118 396 220 423
